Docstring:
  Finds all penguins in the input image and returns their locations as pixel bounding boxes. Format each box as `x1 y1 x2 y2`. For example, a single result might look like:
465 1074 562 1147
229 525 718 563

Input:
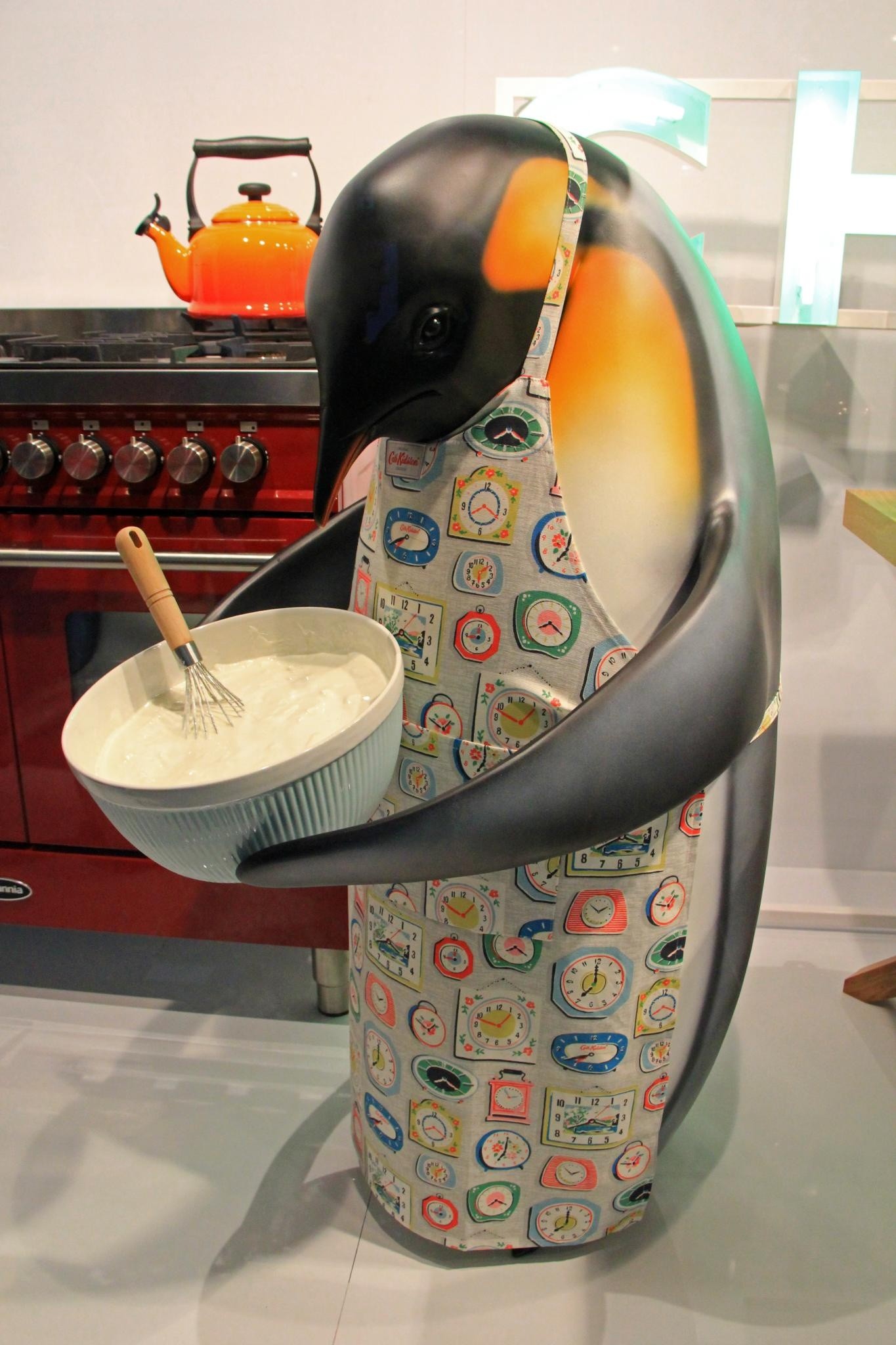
196 119 783 1257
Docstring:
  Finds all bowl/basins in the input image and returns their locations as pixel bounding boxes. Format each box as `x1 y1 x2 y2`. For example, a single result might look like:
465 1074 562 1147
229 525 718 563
61 607 404 884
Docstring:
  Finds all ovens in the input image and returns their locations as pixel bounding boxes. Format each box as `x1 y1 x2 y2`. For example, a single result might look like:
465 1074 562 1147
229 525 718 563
0 405 327 857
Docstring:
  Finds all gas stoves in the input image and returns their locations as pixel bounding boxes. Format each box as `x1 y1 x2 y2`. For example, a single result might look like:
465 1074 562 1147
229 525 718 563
0 331 325 408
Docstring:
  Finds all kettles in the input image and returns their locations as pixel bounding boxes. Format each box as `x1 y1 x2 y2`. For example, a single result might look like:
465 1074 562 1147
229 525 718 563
135 136 322 318
